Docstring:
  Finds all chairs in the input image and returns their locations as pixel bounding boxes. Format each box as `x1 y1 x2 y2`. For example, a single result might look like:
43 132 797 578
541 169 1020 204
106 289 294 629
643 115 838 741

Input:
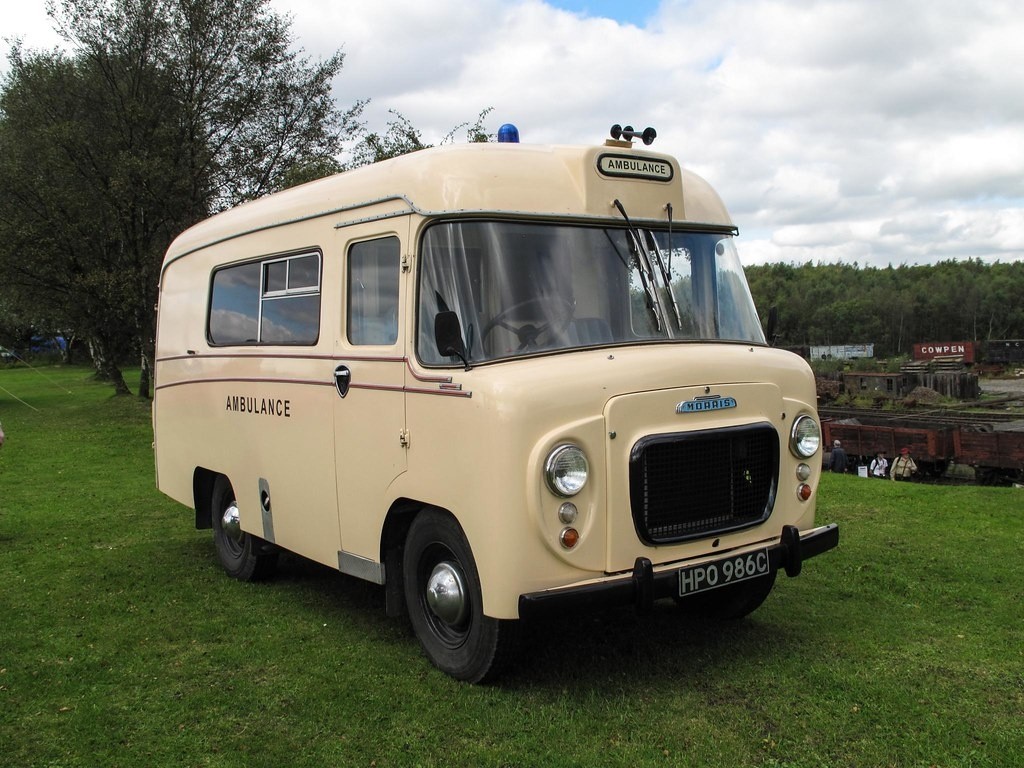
549 318 614 347
386 304 483 351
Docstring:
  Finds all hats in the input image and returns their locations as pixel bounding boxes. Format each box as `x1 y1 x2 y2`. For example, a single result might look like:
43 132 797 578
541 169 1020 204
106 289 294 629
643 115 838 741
834 440 840 446
900 448 909 454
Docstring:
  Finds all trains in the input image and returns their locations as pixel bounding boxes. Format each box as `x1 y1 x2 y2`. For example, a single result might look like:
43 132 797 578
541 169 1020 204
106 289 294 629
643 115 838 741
820 413 1024 487
914 340 1024 366
771 344 874 361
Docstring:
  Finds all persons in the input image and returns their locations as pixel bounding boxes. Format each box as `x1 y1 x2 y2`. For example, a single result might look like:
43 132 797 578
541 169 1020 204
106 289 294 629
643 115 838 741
831 372 872 398
869 451 888 479
829 439 850 473
890 448 918 482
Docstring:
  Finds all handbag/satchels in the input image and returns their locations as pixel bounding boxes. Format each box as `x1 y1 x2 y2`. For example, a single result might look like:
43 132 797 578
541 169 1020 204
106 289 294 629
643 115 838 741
895 474 903 481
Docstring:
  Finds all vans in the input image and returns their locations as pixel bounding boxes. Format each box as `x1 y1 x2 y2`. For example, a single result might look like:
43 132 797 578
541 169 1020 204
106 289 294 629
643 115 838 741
152 122 839 686
